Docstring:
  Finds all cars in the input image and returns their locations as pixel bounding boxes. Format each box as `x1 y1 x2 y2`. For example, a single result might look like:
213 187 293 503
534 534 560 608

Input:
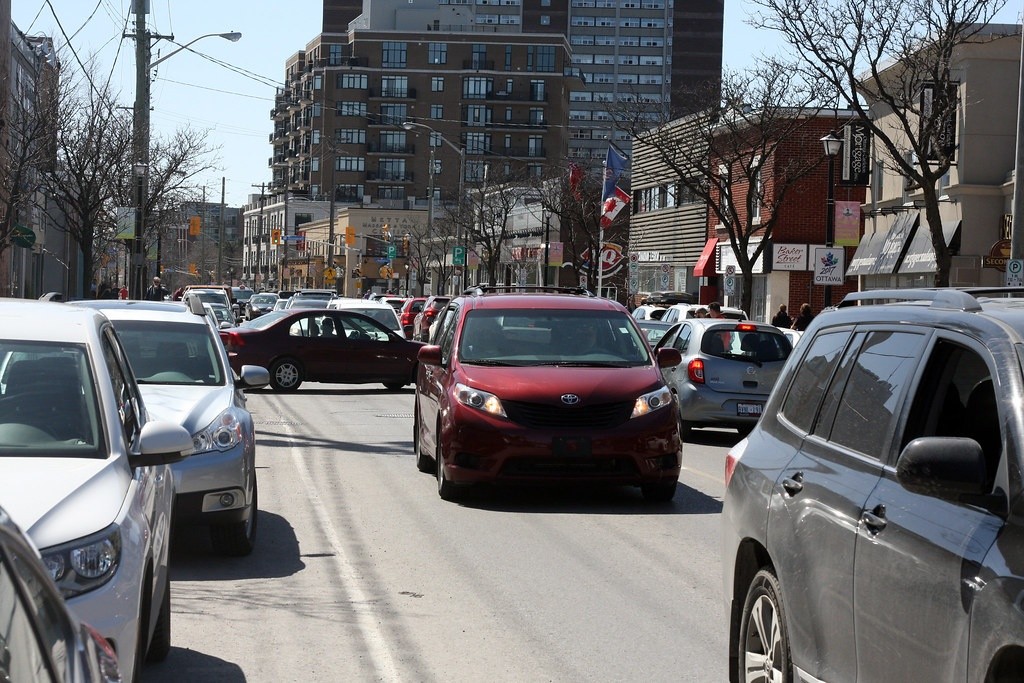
176 283 551 356
0 507 125 683
217 307 429 394
625 301 807 443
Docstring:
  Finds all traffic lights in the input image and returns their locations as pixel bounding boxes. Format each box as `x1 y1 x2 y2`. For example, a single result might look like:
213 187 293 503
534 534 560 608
352 269 361 278
402 235 409 258
271 229 281 245
386 268 394 278
382 223 390 242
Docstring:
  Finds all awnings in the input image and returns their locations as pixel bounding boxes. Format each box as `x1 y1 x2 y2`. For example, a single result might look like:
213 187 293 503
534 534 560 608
693 238 719 277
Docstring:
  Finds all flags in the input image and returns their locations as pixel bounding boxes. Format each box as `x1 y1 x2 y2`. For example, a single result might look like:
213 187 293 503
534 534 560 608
603 144 627 202
570 164 585 201
600 185 631 230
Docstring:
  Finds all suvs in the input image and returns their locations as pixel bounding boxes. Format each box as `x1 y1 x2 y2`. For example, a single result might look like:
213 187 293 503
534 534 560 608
1 289 270 556
0 294 199 683
413 282 685 511
721 287 1024 683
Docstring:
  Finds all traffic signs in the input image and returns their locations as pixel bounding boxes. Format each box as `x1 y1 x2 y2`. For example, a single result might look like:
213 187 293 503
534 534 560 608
453 246 466 265
386 245 396 259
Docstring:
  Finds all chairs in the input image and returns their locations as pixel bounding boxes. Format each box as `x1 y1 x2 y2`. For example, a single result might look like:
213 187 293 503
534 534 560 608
550 321 597 355
2 359 79 411
740 335 760 356
153 342 193 374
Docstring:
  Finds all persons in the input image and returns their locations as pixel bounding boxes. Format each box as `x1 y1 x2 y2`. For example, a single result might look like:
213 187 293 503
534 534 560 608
363 290 371 300
145 277 169 301
172 287 184 301
696 308 710 318
708 302 731 353
790 303 815 331
119 285 127 300
320 318 356 339
111 283 120 300
771 304 793 329
90 279 97 299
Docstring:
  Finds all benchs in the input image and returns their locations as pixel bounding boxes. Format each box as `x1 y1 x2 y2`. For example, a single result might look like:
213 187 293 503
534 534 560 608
708 344 781 360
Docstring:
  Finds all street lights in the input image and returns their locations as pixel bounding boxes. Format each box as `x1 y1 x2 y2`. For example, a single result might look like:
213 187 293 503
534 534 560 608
402 121 466 296
128 28 243 300
198 231 222 285
156 194 167 278
289 196 334 288
818 129 845 307
403 263 411 297
541 207 553 292
250 213 287 291
177 239 205 284
